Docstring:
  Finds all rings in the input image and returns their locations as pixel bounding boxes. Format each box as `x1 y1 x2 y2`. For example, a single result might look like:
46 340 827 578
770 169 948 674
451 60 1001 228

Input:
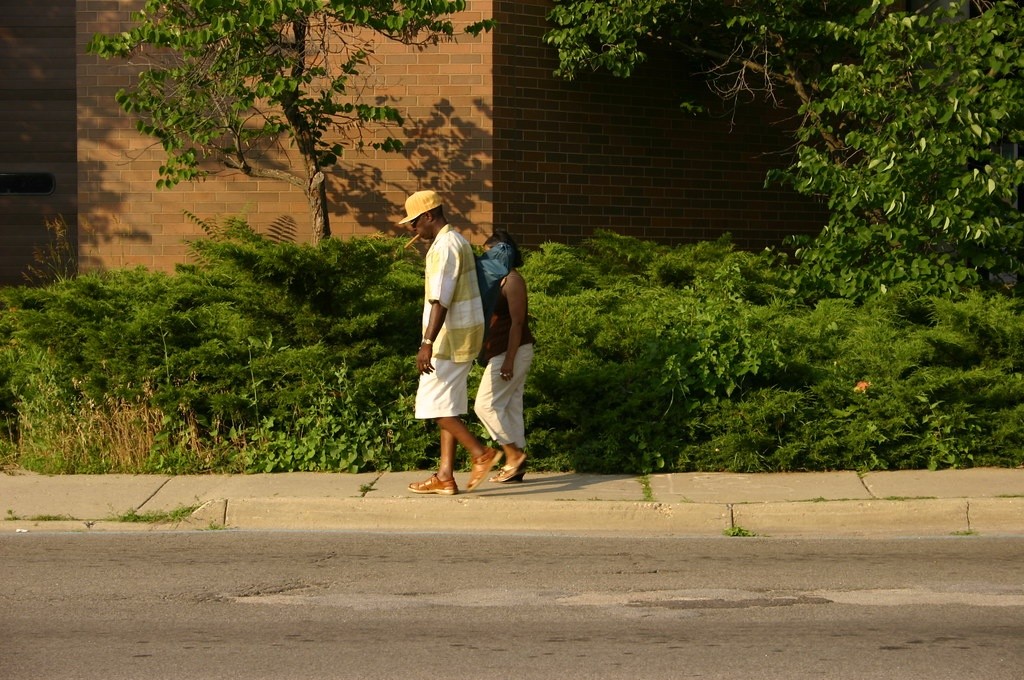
423 364 427 369
427 363 431 366
503 374 506 376
507 373 510 375
500 373 502 375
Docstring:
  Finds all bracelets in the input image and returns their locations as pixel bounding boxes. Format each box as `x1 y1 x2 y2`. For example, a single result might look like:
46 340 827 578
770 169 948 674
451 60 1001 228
421 339 433 345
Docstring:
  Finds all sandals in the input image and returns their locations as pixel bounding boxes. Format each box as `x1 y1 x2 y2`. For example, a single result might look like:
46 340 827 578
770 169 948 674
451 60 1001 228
467 448 504 491
407 473 458 495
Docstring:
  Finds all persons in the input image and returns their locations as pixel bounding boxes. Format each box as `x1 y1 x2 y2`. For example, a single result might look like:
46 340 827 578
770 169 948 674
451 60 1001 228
398 190 504 495
474 232 534 483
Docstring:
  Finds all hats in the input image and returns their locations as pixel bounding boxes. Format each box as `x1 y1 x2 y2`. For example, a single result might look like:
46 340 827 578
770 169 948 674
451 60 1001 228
398 190 442 224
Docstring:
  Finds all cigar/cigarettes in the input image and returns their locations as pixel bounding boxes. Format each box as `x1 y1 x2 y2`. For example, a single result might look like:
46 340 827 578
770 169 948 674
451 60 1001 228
404 234 420 248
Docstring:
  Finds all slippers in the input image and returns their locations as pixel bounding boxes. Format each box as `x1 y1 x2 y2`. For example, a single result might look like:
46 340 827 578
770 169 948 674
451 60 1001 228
497 454 527 481
489 468 526 483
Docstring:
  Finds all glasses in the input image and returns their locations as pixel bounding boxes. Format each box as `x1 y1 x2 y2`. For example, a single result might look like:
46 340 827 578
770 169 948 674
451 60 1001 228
411 217 419 228
